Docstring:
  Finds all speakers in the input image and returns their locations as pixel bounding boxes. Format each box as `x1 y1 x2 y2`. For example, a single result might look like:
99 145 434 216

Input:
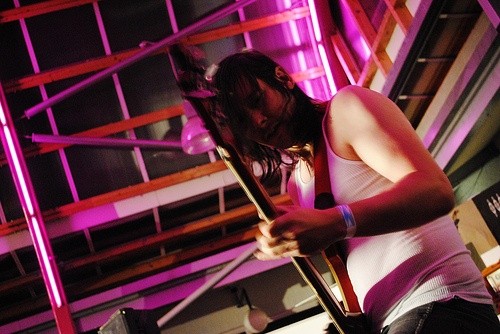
97 307 161 334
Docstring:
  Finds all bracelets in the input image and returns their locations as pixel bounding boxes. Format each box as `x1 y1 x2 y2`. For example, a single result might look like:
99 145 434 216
337 203 358 240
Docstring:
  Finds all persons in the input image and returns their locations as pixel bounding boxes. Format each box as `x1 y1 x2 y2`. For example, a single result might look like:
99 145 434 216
204 47 500 334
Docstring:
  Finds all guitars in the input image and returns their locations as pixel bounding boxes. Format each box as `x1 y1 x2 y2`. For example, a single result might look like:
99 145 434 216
168 40 370 333
